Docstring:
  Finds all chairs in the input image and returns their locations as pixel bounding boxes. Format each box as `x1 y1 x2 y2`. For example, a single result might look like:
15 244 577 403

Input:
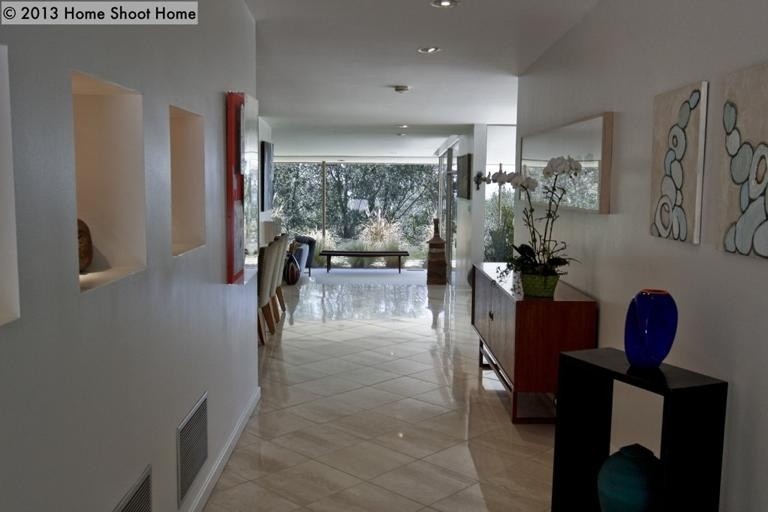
256 233 291 343
283 233 316 277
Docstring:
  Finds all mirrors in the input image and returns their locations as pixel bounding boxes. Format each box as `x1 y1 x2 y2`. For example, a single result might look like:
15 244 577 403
518 110 614 217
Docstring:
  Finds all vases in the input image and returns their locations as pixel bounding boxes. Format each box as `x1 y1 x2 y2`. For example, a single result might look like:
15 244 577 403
521 274 561 299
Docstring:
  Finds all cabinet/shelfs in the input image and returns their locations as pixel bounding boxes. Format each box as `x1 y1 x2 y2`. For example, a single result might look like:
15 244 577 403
469 262 612 424
550 346 729 509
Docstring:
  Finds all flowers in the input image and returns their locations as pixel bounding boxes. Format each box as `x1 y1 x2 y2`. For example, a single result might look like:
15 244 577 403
473 155 584 297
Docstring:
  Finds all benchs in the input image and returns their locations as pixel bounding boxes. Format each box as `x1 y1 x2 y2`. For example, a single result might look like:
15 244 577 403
319 249 410 274
